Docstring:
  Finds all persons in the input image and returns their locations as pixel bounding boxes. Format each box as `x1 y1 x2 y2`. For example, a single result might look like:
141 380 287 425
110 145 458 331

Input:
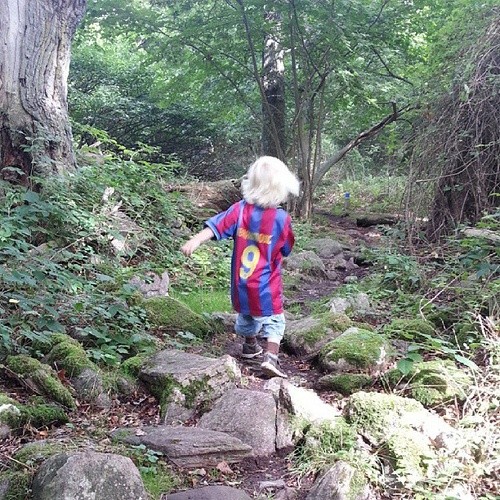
179 156 301 378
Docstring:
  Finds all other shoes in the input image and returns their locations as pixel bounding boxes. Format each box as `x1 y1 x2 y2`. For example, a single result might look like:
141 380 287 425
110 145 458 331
242 342 263 358
261 353 288 378
